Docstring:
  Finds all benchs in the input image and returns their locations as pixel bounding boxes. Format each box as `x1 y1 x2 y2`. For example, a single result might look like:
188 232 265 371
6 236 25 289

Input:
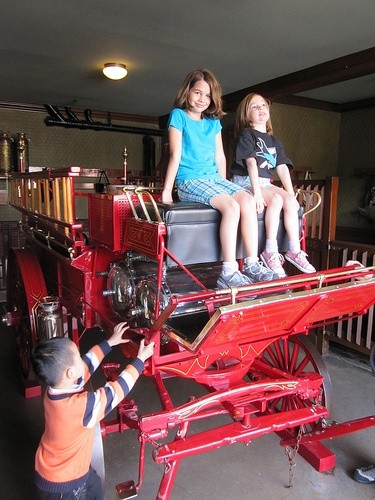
134 202 305 270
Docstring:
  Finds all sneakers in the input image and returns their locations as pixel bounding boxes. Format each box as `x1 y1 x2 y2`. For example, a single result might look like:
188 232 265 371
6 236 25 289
216 269 253 288
284 249 316 273
259 249 287 278
245 261 273 282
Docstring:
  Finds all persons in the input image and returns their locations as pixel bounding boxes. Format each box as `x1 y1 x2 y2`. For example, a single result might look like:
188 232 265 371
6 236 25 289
161 69 279 302
229 94 317 278
34 322 156 500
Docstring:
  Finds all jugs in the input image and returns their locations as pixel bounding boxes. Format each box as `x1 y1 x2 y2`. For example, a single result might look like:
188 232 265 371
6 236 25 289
14 133 29 173
0 133 13 177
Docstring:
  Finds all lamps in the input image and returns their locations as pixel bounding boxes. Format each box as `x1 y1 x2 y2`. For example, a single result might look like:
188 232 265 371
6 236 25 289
103 63 129 80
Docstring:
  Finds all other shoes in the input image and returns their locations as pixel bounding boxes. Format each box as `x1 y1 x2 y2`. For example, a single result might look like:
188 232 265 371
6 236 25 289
352 464 375 484
